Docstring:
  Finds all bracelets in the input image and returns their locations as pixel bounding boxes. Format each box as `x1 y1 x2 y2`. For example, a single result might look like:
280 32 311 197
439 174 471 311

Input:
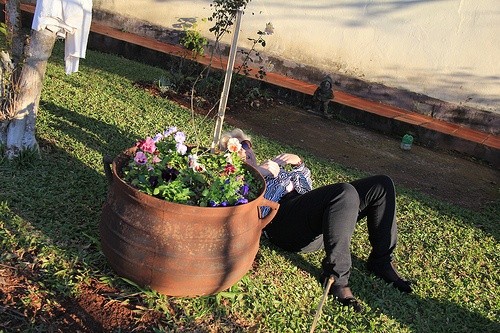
289 160 305 170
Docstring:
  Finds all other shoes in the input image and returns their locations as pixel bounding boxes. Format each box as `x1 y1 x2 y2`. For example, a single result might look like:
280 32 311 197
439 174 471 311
320 273 362 313
366 259 412 293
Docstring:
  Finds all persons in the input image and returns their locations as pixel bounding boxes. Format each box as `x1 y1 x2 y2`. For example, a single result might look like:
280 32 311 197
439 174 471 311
220 128 414 315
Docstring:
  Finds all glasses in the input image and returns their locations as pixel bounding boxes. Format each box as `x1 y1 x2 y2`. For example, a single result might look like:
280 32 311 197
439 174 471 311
240 140 252 150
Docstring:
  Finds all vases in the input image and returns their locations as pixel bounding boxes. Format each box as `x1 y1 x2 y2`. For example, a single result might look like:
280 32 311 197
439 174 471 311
99 144 280 298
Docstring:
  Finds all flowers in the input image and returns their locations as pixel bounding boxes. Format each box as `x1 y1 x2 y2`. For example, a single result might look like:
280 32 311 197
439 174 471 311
122 124 264 207
